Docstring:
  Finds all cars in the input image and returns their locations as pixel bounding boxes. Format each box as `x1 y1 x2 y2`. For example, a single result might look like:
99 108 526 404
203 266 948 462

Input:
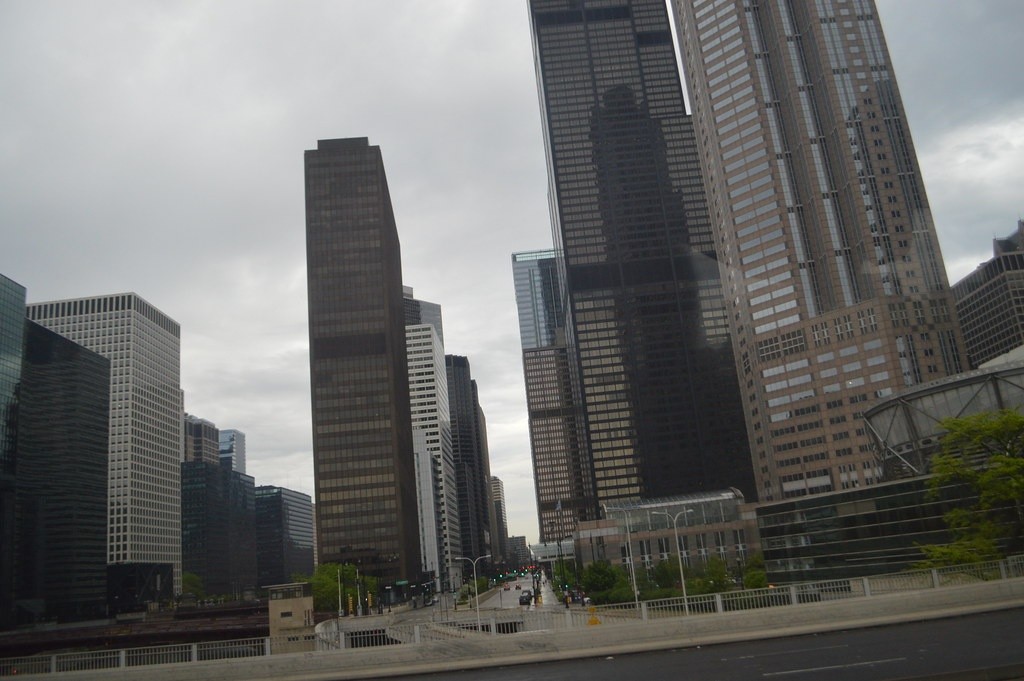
515 584 521 590
504 584 510 591
522 590 532 600
584 597 591 603
519 595 530 605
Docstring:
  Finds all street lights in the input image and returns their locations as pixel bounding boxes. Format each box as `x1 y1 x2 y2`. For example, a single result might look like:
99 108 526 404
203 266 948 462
455 555 491 631
560 534 573 609
737 557 745 590
652 510 693 616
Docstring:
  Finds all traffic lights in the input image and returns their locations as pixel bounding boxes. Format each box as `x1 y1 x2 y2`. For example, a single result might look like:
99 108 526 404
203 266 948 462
368 594 372 606
350 597 353 611
